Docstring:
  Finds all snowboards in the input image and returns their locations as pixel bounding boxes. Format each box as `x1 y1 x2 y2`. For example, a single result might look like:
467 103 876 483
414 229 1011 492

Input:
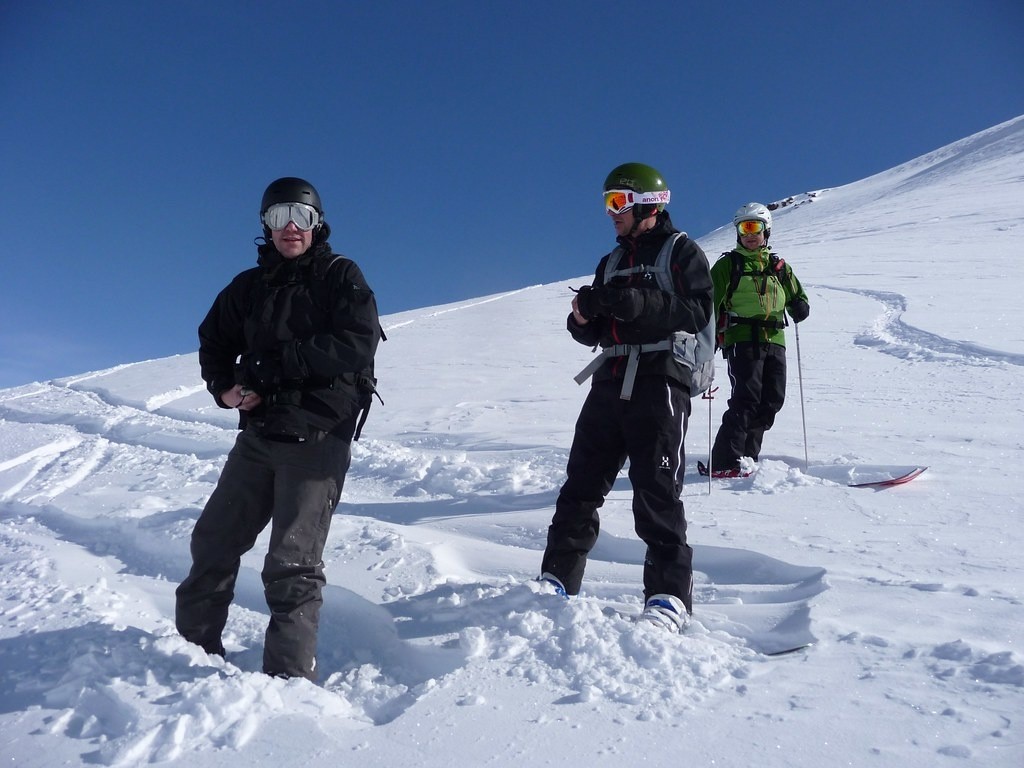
767 643 813 657
695 460 927 490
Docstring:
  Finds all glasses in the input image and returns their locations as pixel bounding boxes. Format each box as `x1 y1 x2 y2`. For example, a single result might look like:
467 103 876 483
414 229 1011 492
736 220 765 238
602 189 671 214
262 202 320 231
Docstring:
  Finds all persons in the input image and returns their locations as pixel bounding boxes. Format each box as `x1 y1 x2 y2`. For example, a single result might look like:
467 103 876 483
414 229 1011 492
175 175 386 682
540 163 715 638
705 202 810 478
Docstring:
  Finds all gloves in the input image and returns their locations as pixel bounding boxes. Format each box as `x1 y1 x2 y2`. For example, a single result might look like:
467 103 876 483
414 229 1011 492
230 335 309 404
599 286 661 323
577 286 633 321
786 298 810 324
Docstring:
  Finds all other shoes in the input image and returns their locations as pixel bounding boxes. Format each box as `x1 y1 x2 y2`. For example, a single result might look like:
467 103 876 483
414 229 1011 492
641 594 688 634
535 572 569 602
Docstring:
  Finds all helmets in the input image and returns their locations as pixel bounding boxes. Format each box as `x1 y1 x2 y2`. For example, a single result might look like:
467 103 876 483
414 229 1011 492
733 202 772 230
604 163 668 220
261 177 325 224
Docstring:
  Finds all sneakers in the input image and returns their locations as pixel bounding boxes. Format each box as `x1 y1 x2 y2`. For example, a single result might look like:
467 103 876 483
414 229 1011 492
713 466 749 478
